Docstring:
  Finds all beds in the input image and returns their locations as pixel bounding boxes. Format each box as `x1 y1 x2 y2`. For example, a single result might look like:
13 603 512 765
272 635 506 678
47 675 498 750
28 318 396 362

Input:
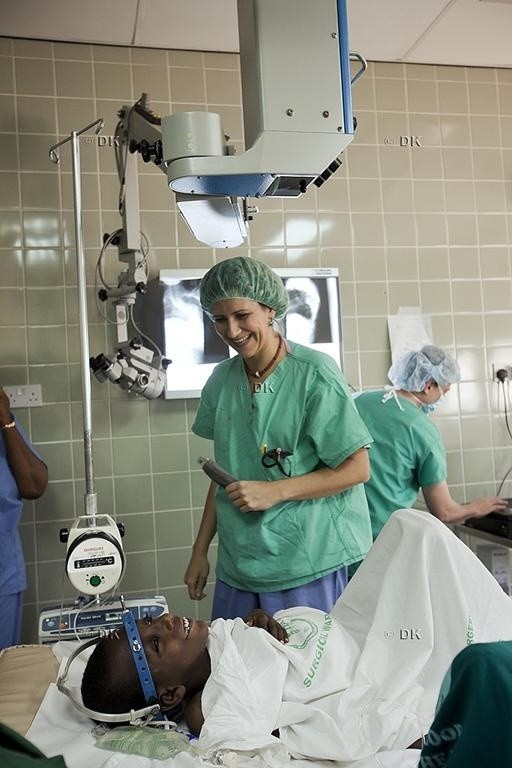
0 644 434 768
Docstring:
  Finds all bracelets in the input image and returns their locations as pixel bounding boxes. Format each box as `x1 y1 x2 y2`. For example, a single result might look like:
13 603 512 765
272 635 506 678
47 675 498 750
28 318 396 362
2 420 16 431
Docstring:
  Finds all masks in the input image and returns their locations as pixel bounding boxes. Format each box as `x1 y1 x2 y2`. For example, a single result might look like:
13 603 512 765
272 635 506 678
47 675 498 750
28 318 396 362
413 386 443 414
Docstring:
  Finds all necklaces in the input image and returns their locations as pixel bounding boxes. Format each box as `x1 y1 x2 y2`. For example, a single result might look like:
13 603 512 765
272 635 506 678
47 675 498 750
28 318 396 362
243 330 283 379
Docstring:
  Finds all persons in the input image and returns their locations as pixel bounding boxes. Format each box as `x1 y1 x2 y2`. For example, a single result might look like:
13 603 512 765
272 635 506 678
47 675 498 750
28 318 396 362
180 254 374 631
0 385 51 652
80 605 288 740
344 344 509 545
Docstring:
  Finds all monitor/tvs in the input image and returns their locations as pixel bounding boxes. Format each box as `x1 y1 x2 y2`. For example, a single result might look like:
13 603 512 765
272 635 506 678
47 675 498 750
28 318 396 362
159 267 344 400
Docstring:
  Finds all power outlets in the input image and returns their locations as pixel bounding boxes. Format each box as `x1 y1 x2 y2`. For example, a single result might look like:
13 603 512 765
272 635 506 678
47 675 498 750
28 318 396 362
3 384 43 409
492 363 512 381
